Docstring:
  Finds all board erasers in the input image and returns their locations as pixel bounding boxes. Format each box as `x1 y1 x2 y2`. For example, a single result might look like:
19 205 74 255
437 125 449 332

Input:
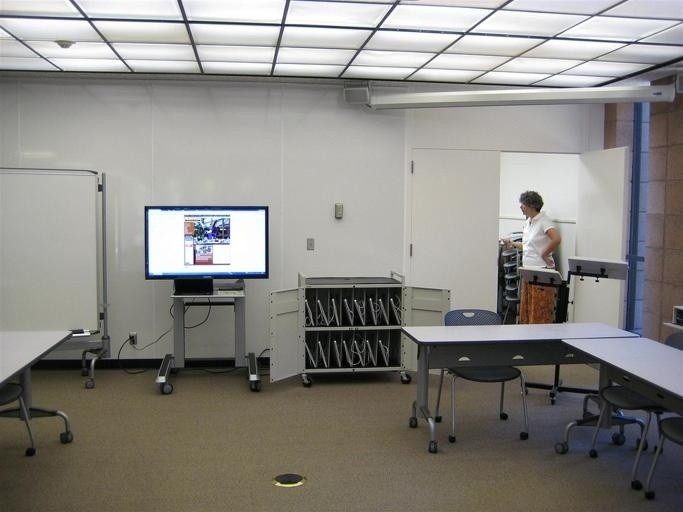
68 329 84 334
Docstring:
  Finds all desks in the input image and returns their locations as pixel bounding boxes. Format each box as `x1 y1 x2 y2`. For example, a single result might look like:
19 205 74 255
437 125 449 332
0 331 74 443
402 323 641 454
555 336 683 462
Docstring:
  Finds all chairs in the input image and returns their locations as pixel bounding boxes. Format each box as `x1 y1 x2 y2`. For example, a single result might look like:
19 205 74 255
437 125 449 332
644 416 683 501
435 308 530 442
0 383 36 457
590 332 683 458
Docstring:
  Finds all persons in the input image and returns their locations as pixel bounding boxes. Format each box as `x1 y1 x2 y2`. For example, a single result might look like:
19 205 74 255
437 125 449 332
502 192 561 325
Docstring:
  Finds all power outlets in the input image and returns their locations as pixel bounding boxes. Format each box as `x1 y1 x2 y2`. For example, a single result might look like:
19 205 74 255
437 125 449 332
128 331 137 345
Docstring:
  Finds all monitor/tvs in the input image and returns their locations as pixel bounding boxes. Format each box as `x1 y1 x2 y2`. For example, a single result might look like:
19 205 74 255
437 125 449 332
144 205 269 279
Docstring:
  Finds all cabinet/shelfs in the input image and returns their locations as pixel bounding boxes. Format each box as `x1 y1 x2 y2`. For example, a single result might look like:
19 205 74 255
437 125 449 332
268 271 451 388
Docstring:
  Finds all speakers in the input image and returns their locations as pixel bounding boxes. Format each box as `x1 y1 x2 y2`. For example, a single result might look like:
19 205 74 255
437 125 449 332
343 87 369 105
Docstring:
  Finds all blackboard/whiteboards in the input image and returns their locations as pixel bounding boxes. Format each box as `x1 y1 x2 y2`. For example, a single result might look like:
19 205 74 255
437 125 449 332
0 168 99 338
